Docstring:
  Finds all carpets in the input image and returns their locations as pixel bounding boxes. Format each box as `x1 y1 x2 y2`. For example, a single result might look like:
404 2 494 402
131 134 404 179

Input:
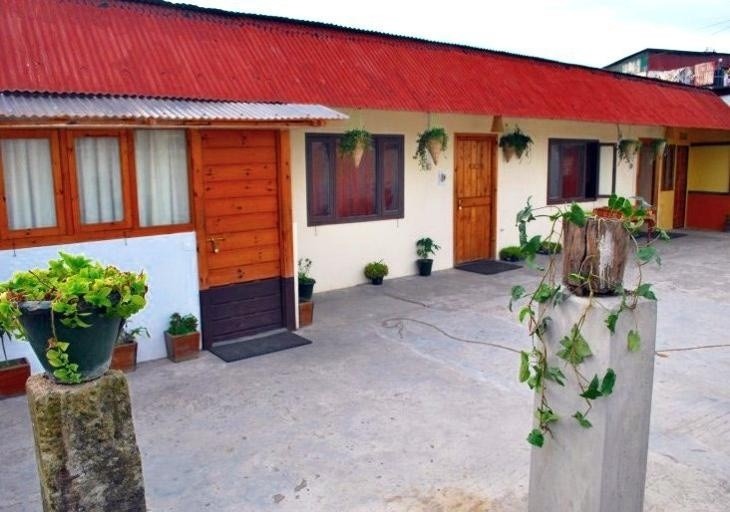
208 330 313 363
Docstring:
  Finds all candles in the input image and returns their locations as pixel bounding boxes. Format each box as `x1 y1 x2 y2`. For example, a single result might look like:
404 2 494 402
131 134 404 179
412 127 450 171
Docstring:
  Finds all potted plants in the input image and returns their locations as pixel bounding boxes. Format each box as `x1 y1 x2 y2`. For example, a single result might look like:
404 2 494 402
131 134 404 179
363 258 389 284
1 251 201 398
498 194 672 449
415 238 442 275
298 258 317 300
616 137 670 170
338 123 373 169
498 122 535 164
